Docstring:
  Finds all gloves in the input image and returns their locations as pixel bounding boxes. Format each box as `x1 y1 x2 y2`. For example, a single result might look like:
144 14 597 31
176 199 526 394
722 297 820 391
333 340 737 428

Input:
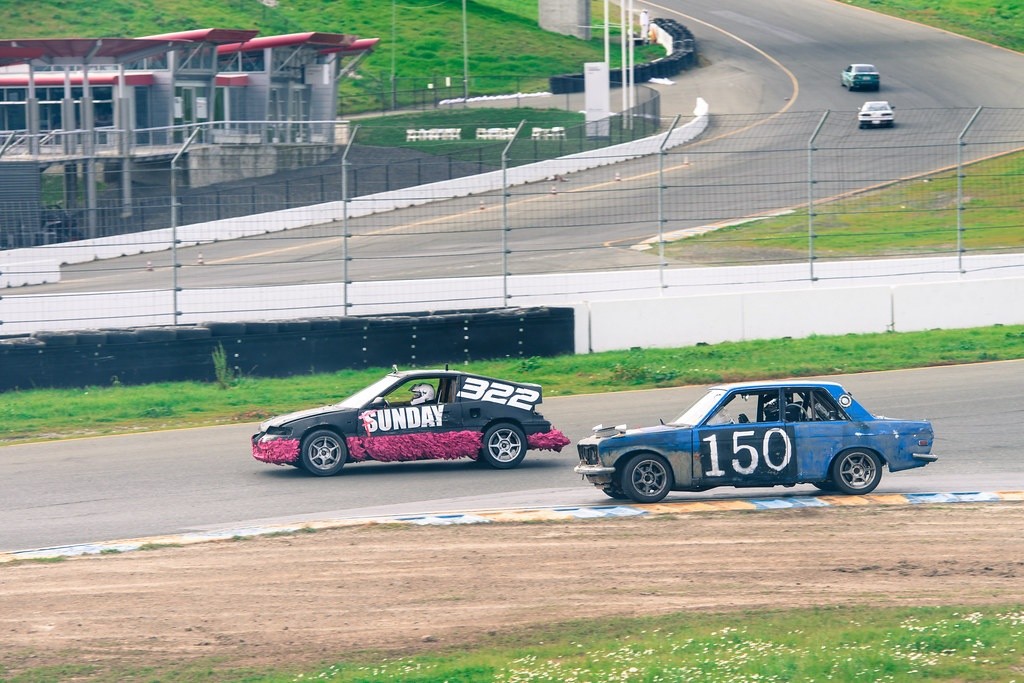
738 414 748 424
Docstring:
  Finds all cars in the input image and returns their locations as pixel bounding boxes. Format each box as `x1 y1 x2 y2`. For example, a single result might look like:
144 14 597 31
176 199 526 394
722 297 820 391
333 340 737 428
250 362 572 477
856 101 896 125
572 379 937 505
840 64 881 91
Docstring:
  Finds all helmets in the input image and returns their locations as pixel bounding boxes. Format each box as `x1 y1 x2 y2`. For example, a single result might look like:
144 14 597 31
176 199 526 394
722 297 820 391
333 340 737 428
408 383 435 405
763 394 790 419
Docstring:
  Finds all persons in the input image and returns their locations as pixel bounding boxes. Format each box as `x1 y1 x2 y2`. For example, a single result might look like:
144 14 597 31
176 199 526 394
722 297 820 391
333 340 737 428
384 383 436 409
738 394 779 423
